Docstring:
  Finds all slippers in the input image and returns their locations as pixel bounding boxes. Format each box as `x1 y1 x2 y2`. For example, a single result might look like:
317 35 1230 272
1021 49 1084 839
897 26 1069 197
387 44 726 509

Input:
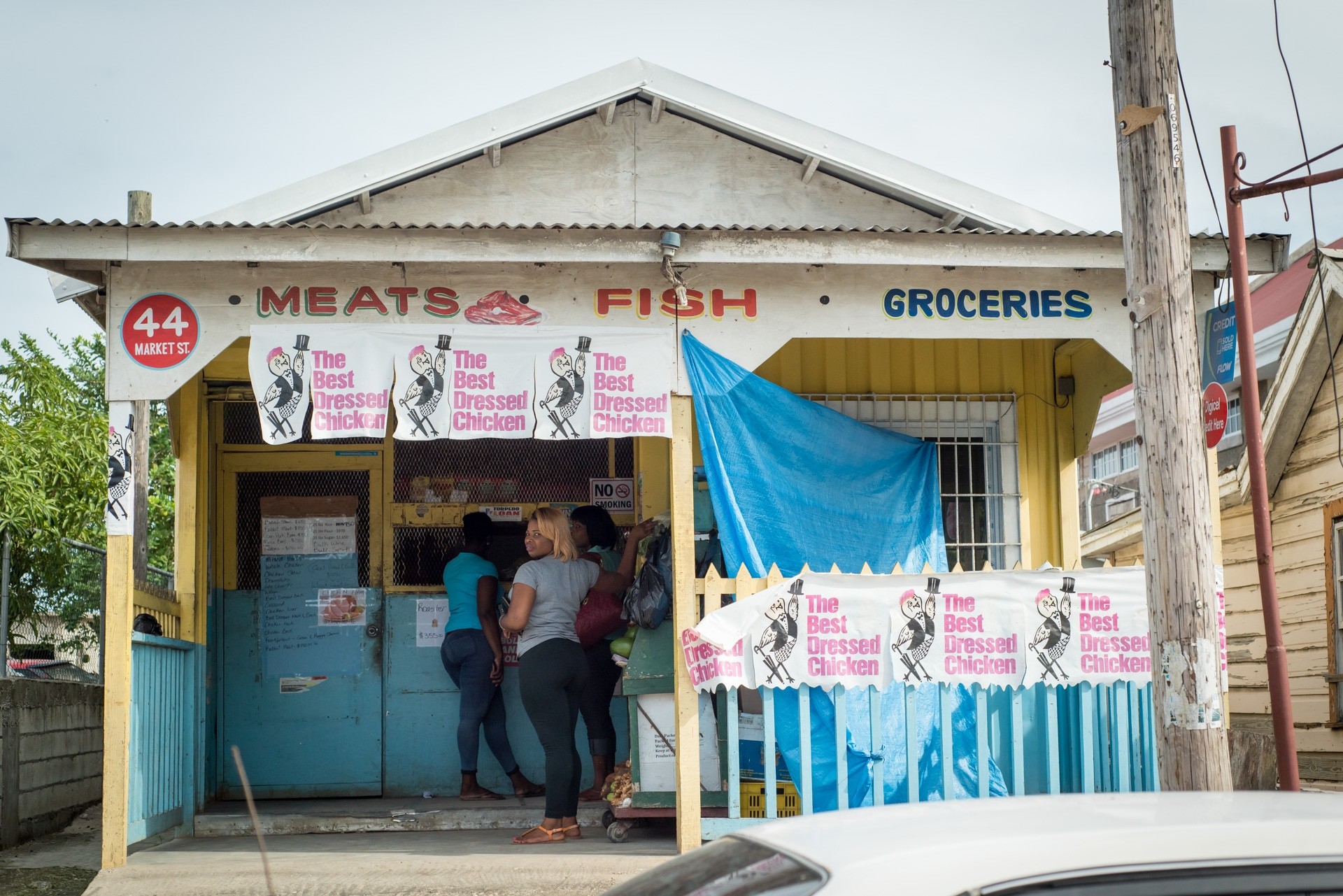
516 786 547 797
461 789 506 800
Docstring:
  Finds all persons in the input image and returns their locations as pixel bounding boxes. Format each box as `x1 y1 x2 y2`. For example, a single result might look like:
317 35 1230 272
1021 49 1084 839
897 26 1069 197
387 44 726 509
440 511 546 801
564 505 636 801
498 506 662 845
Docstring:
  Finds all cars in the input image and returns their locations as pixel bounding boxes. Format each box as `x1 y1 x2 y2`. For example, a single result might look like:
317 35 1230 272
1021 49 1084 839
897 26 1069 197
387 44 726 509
8 658 102 685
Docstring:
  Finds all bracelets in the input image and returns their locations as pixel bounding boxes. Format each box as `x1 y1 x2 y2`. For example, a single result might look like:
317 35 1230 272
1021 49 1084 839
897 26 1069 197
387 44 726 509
499 614 511 632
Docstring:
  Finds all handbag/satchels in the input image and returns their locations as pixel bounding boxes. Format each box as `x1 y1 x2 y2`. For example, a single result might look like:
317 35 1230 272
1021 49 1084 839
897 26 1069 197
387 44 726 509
624 560 668 628
572 588 627 648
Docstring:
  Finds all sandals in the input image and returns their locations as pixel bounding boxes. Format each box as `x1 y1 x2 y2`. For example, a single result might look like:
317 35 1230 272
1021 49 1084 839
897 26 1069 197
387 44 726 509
564 823 582 839
514 825 566 843
